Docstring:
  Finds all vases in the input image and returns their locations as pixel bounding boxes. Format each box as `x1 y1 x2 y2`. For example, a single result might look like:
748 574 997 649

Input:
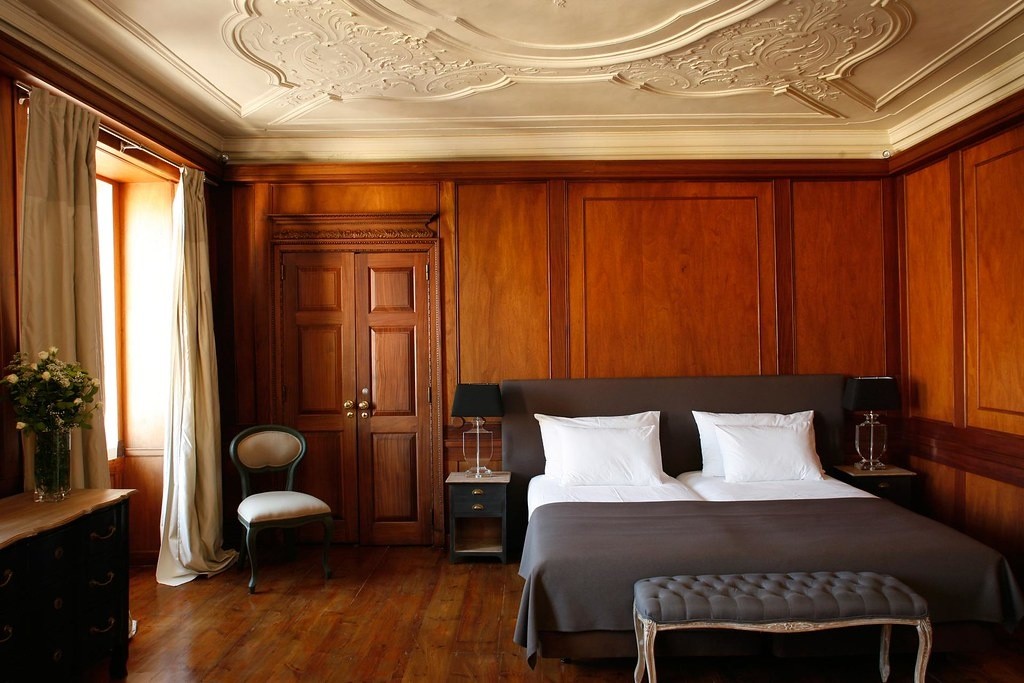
33 425 72 502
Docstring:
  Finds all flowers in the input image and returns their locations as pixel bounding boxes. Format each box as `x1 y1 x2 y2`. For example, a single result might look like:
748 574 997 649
4 347 101 494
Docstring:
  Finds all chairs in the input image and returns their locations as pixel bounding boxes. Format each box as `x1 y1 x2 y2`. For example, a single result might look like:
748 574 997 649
230 424 335 595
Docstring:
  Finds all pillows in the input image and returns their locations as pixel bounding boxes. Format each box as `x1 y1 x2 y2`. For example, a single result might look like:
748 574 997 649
692 409 826 476
715 421 825 482
533 411 664 477
541 423 664 486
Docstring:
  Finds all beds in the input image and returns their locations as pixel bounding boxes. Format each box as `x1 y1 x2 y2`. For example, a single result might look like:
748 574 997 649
501 373 1024 671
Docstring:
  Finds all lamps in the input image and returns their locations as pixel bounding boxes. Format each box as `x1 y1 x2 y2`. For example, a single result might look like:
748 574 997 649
450 383 506 479
842 376 903 470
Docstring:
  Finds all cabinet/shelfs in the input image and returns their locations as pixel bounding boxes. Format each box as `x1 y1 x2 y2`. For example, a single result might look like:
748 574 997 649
445 470 511 564
0 488 140 683
833 464 918 513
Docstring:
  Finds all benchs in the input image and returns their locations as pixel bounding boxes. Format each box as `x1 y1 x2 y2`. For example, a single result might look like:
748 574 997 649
633 573 932 683
